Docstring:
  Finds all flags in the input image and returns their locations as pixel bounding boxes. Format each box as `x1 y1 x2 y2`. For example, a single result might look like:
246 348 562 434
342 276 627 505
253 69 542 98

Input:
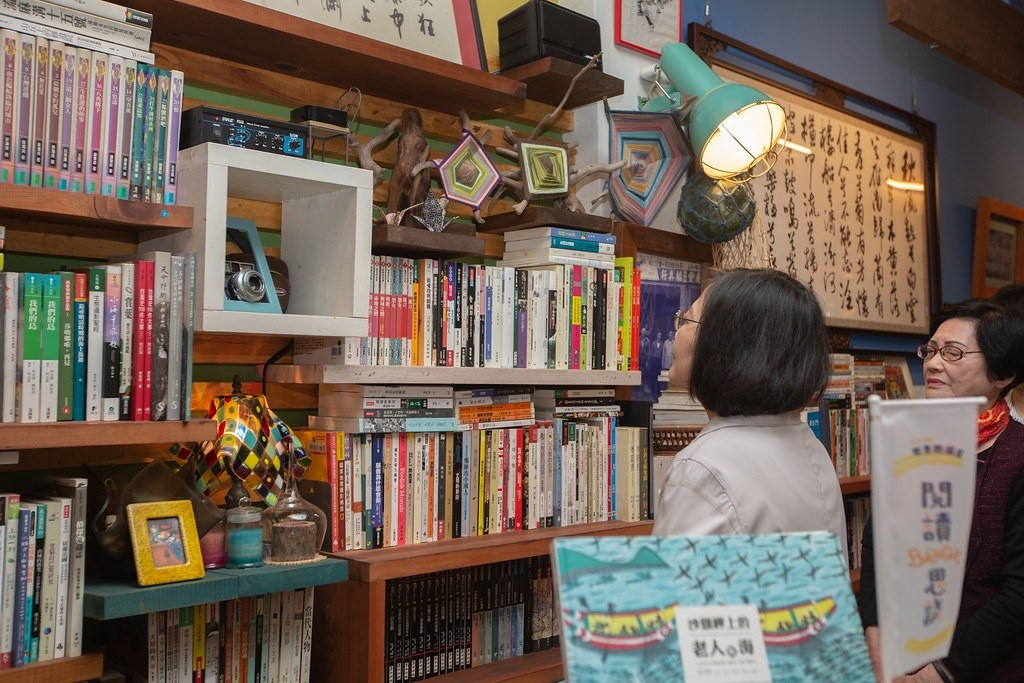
866 393 989 683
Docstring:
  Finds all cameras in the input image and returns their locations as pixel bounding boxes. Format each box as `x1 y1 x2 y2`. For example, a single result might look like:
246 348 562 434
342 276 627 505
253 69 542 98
224 261 267 303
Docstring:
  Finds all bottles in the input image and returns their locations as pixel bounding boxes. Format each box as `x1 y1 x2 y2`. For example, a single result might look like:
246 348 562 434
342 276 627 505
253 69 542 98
202 509 226 568
226 496 264 568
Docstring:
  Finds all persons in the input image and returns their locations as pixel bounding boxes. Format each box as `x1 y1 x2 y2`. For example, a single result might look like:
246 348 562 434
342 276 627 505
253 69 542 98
851 283 1024 683
638 325 674 397
652 265 850 585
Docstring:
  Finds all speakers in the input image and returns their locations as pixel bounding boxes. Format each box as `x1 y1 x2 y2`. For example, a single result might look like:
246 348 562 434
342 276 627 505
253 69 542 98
498 0 604 73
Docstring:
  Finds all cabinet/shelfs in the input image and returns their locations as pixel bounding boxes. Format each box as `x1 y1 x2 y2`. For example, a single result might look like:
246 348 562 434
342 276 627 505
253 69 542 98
0 0 913 683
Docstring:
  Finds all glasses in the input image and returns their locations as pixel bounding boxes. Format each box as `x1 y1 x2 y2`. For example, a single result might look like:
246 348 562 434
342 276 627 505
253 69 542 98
917 344 982 361
673 309 701 332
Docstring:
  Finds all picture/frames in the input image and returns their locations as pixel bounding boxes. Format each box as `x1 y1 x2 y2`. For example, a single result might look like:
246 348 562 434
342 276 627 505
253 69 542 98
971 196 1024 301
614 0 683 58
127 500 205 587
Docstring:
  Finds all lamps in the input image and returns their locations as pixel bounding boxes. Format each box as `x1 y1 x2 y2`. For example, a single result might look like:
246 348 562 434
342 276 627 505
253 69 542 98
169 375 311 509
637 42 787 184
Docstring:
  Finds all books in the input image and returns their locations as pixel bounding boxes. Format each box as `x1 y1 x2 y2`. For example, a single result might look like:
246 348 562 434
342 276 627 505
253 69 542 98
800 353 915 577
548 528 880 683
650 368 710 517
286 226 649 554
104 582 316 683
2 1 199 425
383 554 561 683
1 477 89 668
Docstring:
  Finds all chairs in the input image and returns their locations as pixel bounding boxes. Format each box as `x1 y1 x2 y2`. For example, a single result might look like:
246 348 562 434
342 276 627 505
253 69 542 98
303 87 361 166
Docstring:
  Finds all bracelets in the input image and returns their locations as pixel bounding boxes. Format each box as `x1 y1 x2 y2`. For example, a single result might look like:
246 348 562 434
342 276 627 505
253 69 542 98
931 660 952 683
